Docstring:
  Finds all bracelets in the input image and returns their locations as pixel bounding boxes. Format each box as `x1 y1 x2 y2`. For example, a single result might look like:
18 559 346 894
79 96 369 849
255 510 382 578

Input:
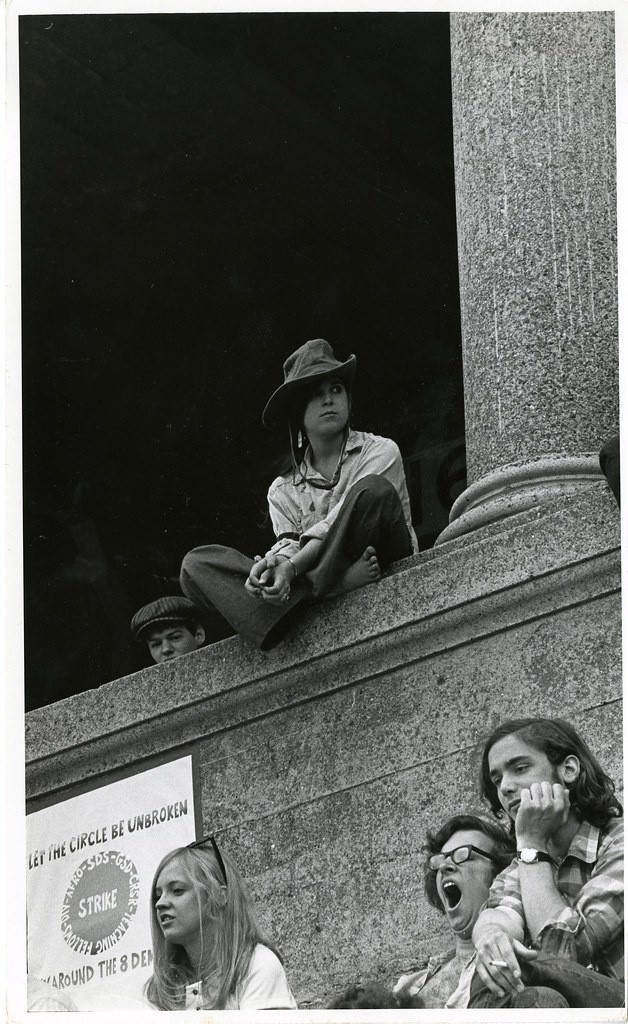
290 560 298 576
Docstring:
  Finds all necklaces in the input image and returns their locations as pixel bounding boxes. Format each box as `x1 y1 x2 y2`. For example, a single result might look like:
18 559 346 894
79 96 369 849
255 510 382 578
190 940 213 969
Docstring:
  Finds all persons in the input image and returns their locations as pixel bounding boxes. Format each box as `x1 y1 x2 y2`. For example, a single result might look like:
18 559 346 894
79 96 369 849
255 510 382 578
325 979 426 1008
180 339 418 649
138 834 299 1011
467 716 625 1008
390 816 514 1008
132 595 206 663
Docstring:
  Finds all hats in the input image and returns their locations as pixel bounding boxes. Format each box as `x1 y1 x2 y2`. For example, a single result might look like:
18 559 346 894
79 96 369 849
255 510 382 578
130 595 200 639
262 339 357 427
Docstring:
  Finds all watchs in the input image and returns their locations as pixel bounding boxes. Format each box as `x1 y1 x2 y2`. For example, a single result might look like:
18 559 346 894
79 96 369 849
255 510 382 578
517 848 554 866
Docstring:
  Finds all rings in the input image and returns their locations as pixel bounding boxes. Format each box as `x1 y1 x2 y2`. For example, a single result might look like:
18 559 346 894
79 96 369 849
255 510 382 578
283 594 290 600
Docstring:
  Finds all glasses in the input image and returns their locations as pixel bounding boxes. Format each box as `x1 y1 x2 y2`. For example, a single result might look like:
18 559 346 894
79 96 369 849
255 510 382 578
428 844 501 871
185 835 227 884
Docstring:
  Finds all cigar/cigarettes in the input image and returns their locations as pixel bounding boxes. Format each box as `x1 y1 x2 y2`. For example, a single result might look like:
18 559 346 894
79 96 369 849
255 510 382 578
489 961 507 968
